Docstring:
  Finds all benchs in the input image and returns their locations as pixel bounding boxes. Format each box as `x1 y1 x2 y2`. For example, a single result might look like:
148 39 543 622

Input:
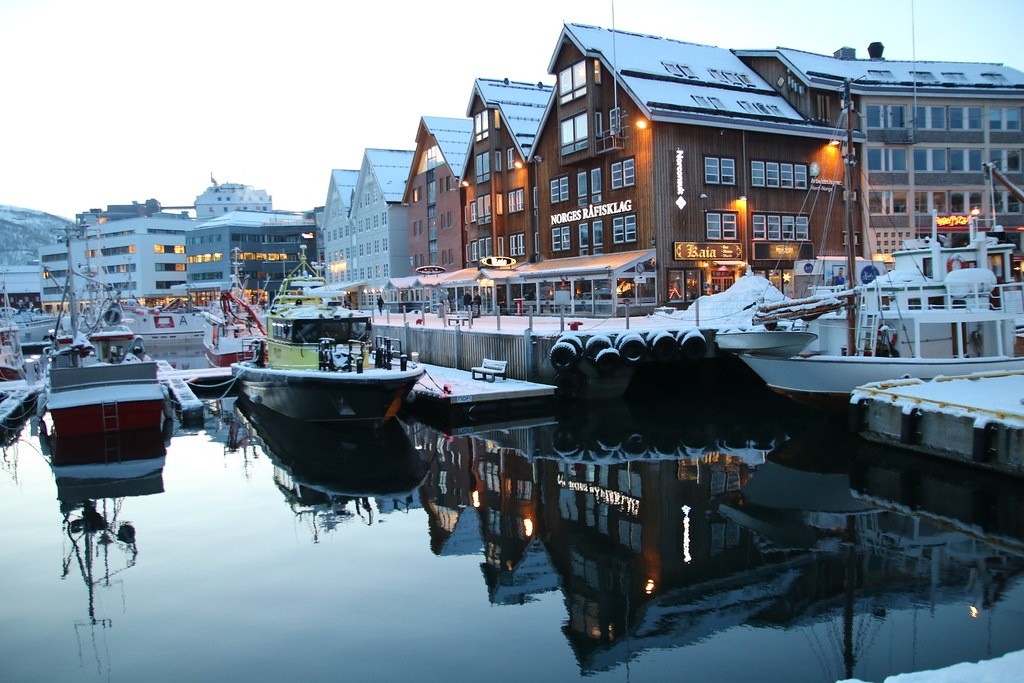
446 311 473 328
471 358 509 383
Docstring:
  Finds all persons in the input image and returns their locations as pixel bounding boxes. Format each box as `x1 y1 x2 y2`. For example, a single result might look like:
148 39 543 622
972 324 983 358
464 290 471 311
834 268 845 285
473 292 481 315
377 297 384 316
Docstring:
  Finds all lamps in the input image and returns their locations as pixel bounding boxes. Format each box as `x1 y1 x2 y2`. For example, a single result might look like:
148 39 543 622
534 155 542 164
740 196 747 200
700 194 708 198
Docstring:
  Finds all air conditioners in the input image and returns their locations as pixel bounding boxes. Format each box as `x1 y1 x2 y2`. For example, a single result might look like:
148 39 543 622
841 145 855 156
841 99 853 110
844 234 857 243
842 191 856 202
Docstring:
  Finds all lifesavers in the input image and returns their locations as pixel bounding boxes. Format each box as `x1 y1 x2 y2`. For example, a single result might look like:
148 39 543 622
946 254 967 273
877 323 898 351
553 424 711 465
550 328 707 375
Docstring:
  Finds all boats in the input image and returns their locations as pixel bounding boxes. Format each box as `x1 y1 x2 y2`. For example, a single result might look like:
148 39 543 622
204 247 268 368
36 214 164 439
233 244 426 424
718 77 1024 392
